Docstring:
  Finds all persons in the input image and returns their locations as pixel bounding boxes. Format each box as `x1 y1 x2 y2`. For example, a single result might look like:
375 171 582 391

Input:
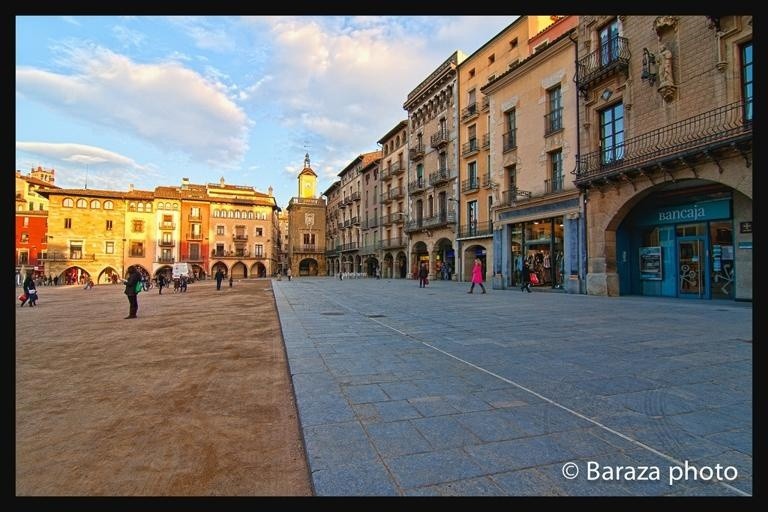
519 260 533 294
466 258 487 294
15 258 455 322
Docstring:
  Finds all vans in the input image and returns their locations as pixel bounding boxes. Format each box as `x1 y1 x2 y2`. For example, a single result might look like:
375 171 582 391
172 262 195 284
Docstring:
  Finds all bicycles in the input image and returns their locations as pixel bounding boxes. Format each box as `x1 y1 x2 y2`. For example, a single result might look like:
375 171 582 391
141 276 170 292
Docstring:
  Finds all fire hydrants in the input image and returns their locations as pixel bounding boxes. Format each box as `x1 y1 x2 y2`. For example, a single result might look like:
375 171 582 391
276 273 282 281
228 276 234 287
